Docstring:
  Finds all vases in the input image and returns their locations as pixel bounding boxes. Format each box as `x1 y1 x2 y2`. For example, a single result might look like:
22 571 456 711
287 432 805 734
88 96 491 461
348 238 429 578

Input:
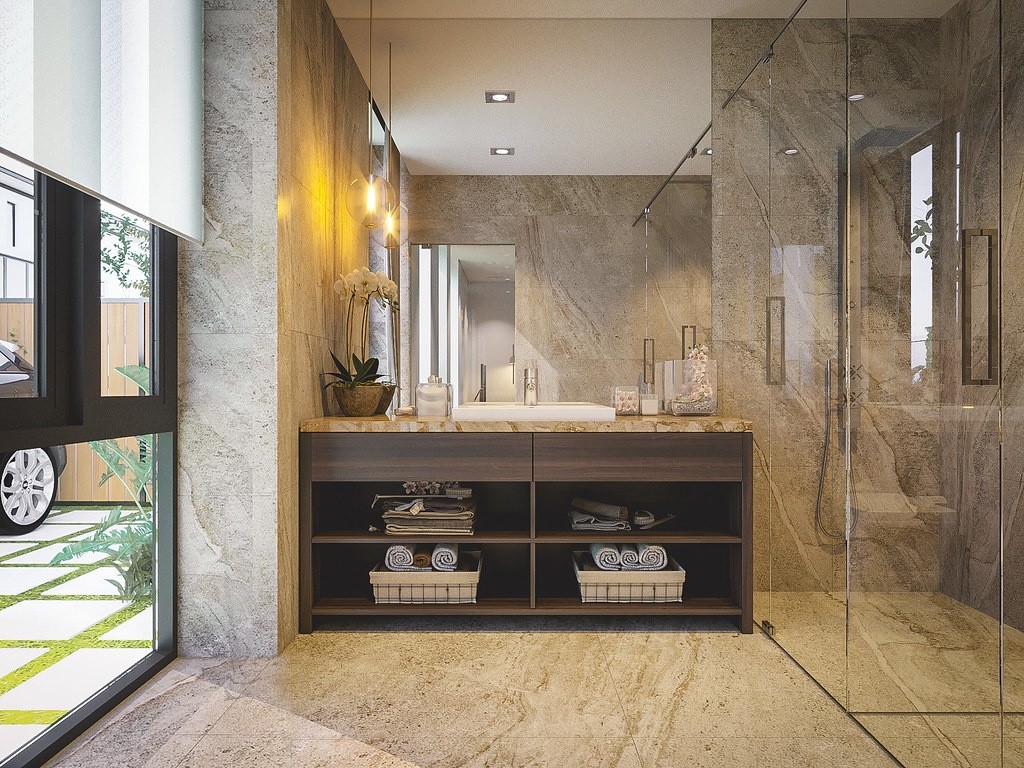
332 385 383 418
374 384 397 414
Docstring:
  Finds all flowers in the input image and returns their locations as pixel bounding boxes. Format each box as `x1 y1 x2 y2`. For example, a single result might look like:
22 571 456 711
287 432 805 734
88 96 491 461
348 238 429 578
402 479 461 495
319 266 393 391
687 342 712 402
360 266 403 391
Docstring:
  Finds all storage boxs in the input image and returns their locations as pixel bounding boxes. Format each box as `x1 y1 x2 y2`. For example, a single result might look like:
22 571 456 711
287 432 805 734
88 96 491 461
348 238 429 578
570 549 686 603
368 549 485 605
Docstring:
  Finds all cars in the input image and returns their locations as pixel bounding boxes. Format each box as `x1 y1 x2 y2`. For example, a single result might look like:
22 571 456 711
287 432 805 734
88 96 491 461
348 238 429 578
0 338 69 536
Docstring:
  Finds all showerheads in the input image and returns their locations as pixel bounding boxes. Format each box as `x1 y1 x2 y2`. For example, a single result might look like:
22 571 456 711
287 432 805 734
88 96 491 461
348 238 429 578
853 125 923 151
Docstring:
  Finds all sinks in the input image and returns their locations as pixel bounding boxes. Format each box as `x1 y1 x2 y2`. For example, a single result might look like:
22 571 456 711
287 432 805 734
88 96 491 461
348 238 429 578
454 401 618 424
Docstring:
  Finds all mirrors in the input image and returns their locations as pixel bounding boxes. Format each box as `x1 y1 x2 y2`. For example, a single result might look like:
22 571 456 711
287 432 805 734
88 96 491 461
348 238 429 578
408 241 516 407
335 18 713 415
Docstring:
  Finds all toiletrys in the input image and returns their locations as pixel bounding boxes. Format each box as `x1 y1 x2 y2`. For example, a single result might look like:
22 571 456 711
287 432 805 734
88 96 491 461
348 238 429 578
639 382 658 416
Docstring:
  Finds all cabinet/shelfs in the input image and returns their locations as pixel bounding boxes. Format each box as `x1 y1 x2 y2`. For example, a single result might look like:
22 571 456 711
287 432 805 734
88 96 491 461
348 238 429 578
310 432 743 618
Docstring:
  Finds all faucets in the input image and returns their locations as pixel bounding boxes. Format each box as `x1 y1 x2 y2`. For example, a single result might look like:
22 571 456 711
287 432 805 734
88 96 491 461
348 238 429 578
523 368 538 405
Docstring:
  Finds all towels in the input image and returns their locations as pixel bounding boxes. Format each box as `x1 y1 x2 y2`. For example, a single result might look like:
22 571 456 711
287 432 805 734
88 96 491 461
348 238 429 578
568 496 632 532
383 544 461 574
381 499 477 536
587 544 670 571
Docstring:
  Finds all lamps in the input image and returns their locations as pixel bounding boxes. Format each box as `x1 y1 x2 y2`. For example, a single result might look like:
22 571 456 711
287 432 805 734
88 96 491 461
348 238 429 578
344 0 398 229
369 40 413 250
